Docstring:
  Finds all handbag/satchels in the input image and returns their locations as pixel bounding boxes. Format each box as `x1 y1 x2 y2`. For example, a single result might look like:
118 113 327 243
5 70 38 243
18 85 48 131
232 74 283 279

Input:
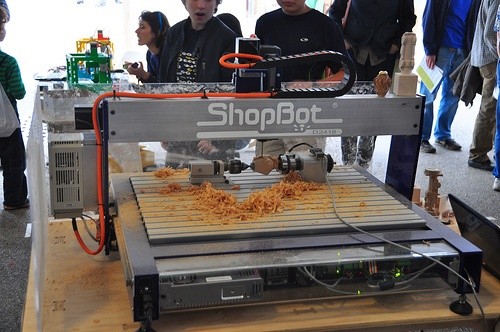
0 83 21 139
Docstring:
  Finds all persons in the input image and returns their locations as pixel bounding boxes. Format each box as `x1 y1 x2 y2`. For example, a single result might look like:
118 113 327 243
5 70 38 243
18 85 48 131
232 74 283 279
123 0 500 192
0 6 31 210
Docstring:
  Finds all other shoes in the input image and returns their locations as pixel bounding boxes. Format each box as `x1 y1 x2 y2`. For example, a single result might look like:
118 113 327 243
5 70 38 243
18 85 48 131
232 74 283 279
434 138 462 150
467 158 495 171
420 140 436 153
493 178 500 191
4 198 30 209
